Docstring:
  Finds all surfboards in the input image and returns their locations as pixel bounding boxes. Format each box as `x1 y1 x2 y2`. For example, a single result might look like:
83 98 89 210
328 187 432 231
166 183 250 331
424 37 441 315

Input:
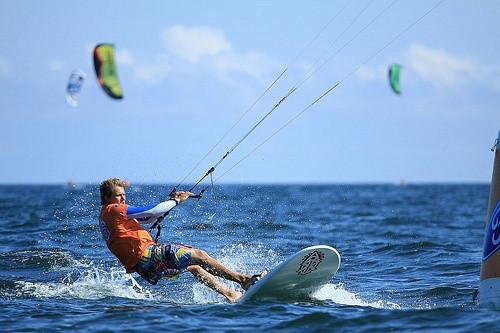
232 245 341 305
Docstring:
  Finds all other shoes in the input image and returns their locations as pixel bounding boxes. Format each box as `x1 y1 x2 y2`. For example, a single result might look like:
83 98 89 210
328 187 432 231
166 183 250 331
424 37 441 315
239 267 267 291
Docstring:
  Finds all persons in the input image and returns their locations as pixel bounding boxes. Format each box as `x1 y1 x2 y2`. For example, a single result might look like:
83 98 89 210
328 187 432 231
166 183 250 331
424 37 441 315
98 177 269 302
